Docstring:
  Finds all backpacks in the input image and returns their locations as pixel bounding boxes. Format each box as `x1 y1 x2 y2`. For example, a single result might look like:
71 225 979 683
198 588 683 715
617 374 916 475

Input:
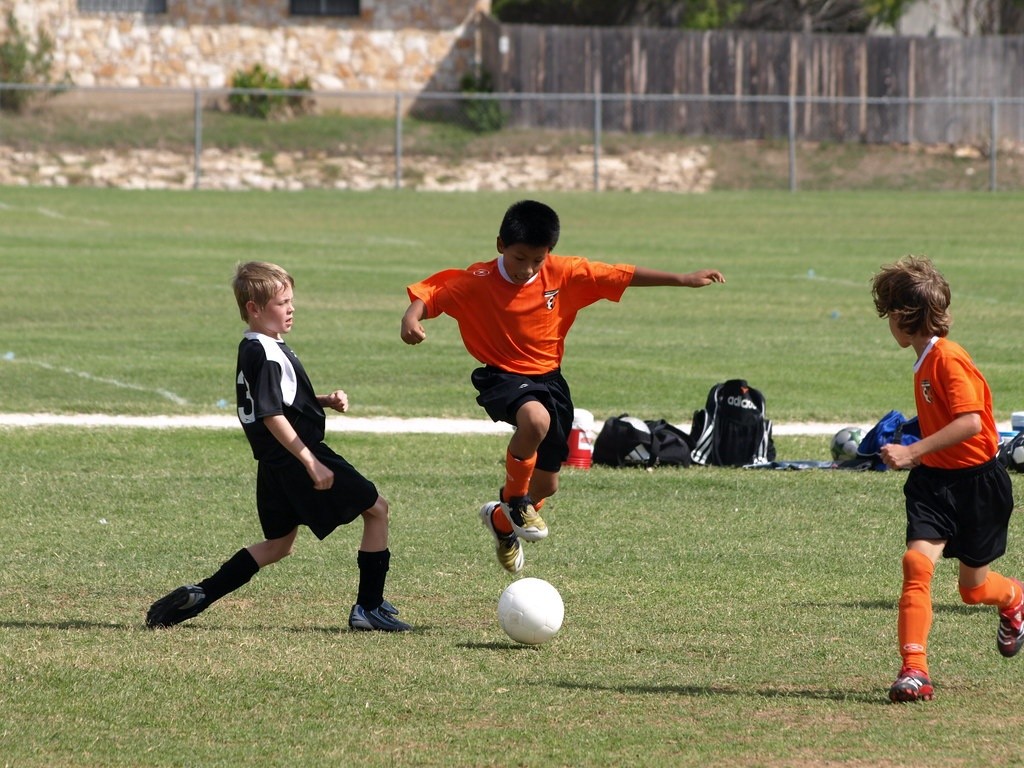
689 377 776 466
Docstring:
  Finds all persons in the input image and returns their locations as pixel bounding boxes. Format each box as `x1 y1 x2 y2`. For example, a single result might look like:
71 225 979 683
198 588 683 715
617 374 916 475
146 263 411 630
871 252 1023 699
401 200 725 571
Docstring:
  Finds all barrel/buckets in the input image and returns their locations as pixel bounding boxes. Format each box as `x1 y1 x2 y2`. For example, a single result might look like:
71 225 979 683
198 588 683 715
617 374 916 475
1011 412 1024 434
561 429 591 469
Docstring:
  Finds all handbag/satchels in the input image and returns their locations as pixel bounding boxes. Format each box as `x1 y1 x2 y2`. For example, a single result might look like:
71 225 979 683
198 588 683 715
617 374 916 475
855 408 920 471
593 413 692 467
998 430 1024 473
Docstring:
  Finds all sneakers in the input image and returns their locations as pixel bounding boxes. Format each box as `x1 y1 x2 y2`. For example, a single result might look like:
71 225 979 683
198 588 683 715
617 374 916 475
146 584 206 628
480 501 524 573
997 577 1024 657
500 486 548 541
889 667 934 701
349 600 414 631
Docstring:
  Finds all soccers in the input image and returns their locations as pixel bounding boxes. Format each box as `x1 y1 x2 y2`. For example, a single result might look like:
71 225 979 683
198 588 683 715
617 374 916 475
830 426 868 465
497 578 565 645
1009 433 1024 473
